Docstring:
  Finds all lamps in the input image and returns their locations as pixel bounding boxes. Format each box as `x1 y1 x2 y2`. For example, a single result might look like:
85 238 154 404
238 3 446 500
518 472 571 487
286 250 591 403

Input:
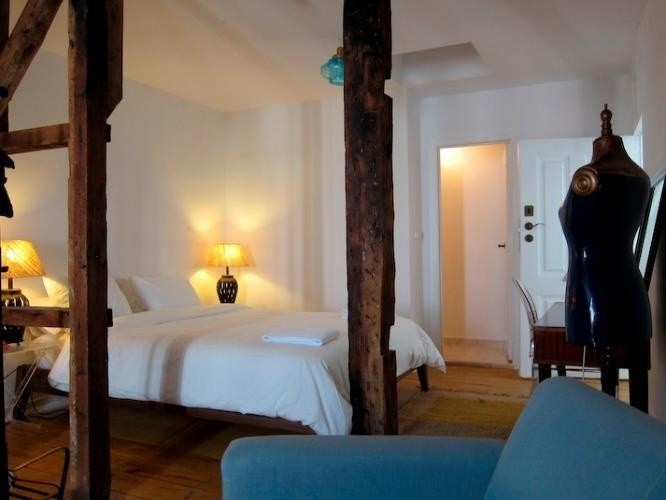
204 242 256 304
1 240 46 344
319 46 345 86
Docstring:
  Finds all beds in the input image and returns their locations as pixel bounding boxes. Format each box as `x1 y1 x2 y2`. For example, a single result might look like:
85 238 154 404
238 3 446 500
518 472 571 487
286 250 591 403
13 273 447 435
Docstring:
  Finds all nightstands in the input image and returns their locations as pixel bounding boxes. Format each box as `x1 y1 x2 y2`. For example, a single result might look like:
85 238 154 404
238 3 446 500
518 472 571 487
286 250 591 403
4 345 36 423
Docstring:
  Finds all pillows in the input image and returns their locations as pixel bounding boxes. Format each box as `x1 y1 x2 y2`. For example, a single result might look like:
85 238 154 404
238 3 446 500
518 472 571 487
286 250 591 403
132 274 202 310
42 272 134 331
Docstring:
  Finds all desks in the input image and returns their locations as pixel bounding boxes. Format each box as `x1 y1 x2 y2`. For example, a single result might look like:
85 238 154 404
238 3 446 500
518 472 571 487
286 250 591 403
532 301 650 414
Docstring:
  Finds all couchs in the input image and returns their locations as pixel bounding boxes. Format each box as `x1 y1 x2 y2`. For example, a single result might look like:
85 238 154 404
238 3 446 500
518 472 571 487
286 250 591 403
221 376 666 500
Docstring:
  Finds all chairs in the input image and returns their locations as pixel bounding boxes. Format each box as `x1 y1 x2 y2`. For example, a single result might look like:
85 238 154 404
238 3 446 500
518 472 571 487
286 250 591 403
511 277 620 400
